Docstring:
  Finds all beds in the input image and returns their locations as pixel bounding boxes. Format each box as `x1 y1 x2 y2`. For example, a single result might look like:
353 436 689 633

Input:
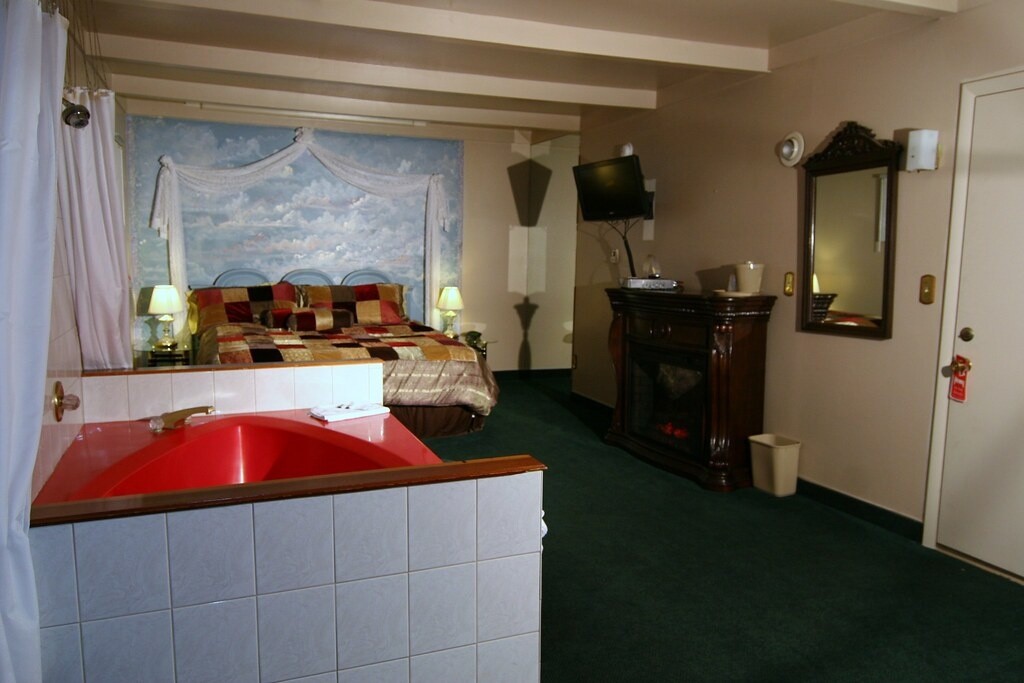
188 268 500 440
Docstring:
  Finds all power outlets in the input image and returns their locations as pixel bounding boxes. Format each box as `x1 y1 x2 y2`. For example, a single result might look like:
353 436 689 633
611 249 619 264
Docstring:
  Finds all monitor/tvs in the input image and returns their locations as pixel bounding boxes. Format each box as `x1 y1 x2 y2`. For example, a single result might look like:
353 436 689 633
573 154 649 222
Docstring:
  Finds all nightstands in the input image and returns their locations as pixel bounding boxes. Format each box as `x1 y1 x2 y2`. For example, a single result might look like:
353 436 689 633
457 336 498 362
134 342 192 367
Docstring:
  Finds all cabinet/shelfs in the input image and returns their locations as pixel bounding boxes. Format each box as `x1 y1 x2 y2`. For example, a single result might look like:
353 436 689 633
600 286 778 494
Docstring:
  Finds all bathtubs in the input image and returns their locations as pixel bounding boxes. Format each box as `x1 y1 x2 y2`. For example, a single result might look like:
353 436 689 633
35 406 445 505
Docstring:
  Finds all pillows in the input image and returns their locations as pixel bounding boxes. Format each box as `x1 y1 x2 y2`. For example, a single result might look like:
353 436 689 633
286 310 355 331
185 281 297 336
295 282 410 327
266 308 318 329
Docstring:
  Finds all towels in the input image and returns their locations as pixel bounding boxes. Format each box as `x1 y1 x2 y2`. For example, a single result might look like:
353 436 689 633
310 403 391 423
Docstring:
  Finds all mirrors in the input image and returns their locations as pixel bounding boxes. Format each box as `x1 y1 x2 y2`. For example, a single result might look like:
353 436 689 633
801 120 904 340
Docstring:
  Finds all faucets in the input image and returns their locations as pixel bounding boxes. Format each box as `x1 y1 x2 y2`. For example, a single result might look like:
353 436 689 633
161 405 215 427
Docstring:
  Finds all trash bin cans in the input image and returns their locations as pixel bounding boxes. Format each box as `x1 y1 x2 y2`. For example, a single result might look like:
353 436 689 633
748 432 802 497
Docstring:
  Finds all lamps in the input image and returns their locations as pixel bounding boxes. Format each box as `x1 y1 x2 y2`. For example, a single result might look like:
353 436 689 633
436 287 465 338
779 131 804 167
147 285 183 348
